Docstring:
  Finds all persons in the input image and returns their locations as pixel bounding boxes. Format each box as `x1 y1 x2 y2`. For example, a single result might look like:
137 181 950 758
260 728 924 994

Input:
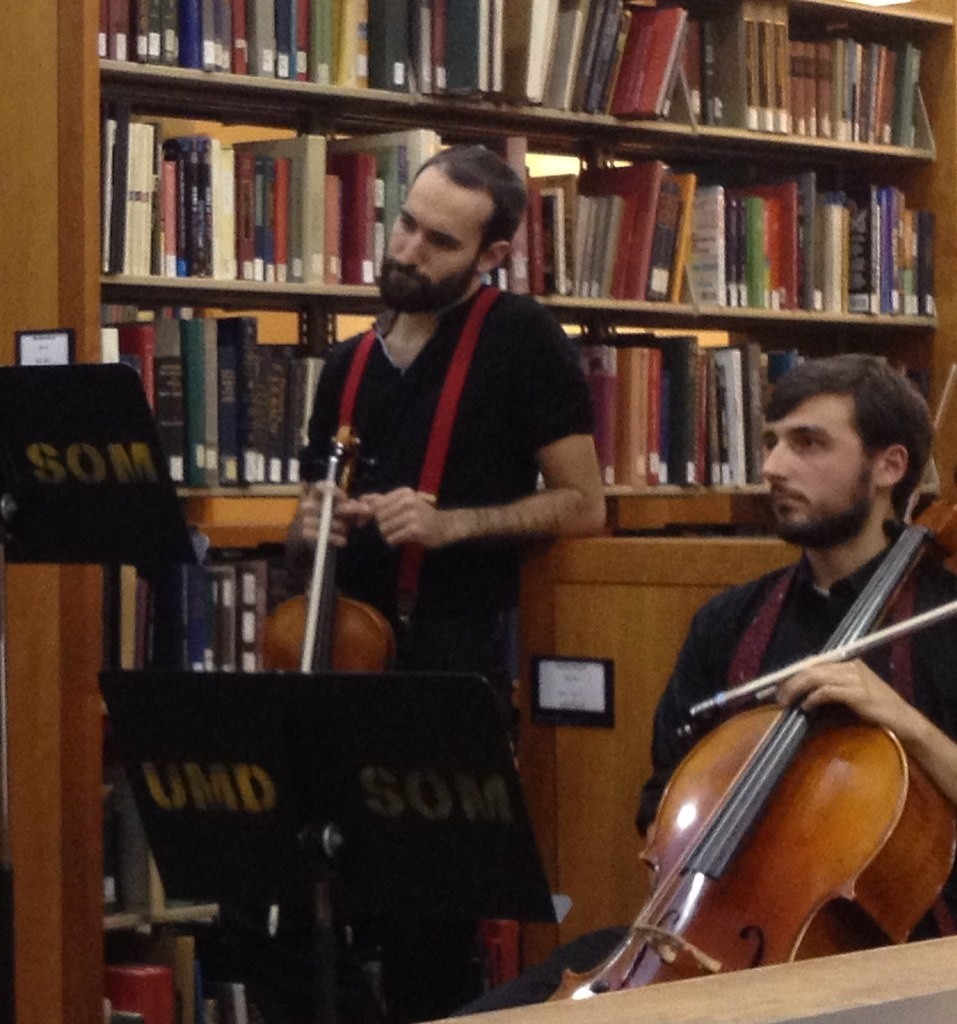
285 148 605 1023
456 354 957 1024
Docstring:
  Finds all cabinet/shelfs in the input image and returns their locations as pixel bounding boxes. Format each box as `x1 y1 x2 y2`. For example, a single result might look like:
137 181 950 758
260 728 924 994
0 1 957 1024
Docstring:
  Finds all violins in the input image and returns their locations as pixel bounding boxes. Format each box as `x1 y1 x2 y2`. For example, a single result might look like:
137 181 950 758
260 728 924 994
255 430 394 670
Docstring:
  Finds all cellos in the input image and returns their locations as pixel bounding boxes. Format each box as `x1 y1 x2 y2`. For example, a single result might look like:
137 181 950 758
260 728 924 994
542 481 956 1006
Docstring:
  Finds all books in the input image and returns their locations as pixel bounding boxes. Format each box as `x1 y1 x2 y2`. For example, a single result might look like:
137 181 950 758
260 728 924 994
99 0 920 147
104 770 248 1024
121 559 264 670
102 306 916 485
102 104 934 315
